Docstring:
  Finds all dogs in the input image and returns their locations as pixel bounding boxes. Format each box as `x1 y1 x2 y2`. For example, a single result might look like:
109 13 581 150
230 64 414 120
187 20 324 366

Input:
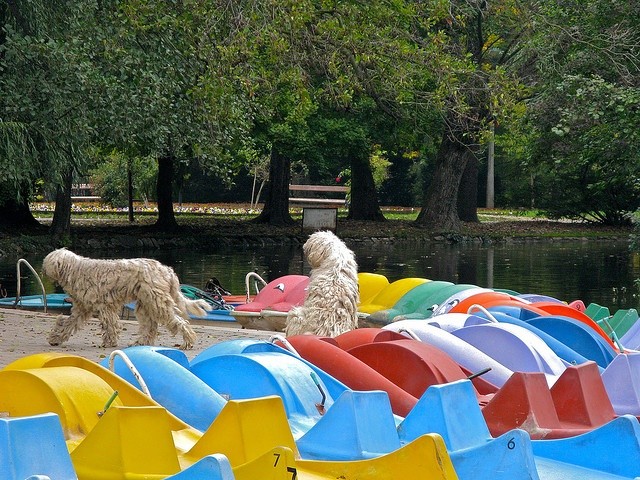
276 232 361 345
39 228 208 350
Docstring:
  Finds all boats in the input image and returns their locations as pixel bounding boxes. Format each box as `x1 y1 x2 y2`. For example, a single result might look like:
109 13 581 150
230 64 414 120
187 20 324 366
0 272 640 478
